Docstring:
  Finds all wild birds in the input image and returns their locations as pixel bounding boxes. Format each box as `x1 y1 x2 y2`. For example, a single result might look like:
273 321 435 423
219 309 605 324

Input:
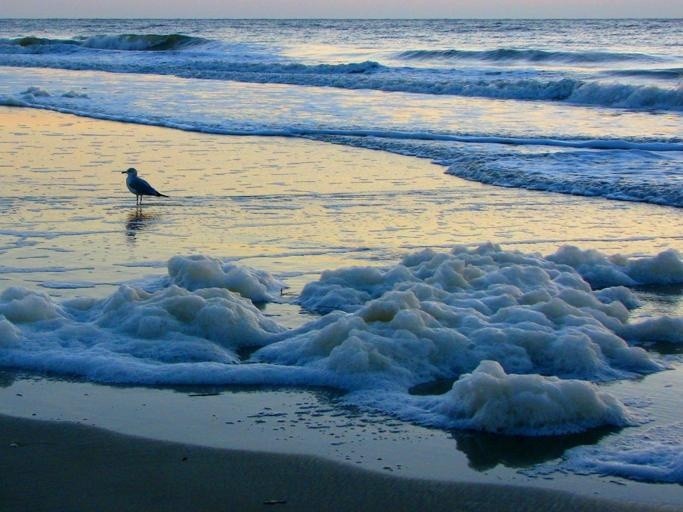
121 167 170 205
